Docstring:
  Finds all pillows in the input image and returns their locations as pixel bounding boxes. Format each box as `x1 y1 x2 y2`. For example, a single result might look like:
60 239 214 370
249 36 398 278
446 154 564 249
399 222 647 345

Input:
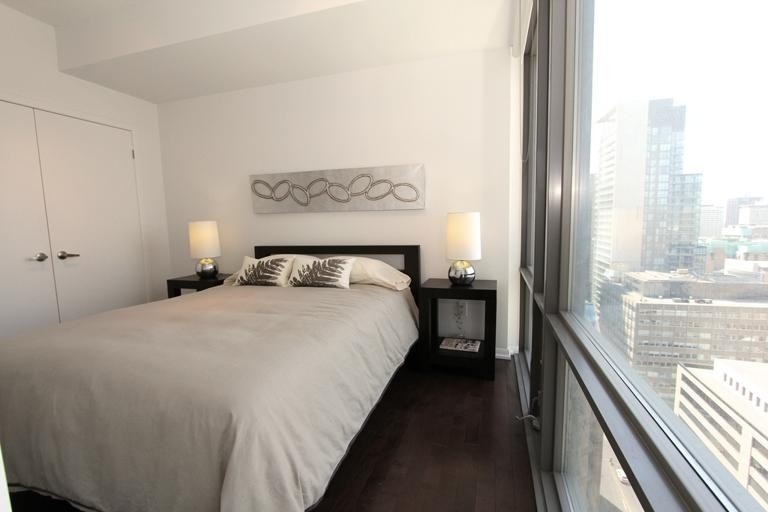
321 257 412 291
232 256 296 287
288 257 356 289
235 254 321 284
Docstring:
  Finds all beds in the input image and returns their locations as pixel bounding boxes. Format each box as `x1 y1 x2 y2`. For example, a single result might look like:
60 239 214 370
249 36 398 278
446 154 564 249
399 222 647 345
0 245 422 512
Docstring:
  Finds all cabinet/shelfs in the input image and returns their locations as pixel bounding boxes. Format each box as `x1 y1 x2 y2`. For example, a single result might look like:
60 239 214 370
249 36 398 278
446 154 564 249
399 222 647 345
0 100 147 337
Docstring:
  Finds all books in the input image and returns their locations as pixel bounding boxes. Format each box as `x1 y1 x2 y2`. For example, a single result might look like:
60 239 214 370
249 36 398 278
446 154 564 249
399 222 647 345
440 337 481 352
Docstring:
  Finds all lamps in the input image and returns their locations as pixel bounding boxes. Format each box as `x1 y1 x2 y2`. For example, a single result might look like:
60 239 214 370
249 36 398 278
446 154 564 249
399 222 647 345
188 221 222 278
444 212 482 285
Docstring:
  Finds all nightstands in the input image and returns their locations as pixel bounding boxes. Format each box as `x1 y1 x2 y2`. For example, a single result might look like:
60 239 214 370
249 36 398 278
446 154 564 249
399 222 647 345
420 277 497 381
167 273 233 298
